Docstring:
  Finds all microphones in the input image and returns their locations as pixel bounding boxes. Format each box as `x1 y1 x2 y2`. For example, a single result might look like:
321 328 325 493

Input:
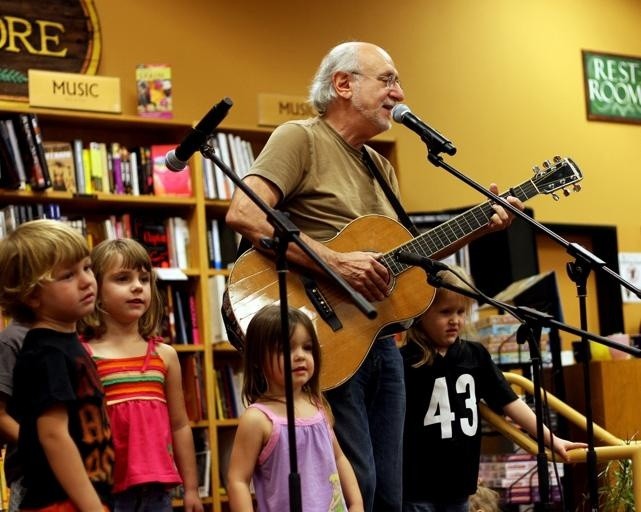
393 249 449 271
165 98 234 174
390 103 457 156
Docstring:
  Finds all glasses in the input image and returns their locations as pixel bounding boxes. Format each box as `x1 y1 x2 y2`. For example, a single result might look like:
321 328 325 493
356 74 402 90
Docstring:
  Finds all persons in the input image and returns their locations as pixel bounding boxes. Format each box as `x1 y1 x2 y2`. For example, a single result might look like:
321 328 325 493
0 218 117 512
225 40 526 511
224 302 365 512
78 235 209 512
0 315 30 512
397 263 590 511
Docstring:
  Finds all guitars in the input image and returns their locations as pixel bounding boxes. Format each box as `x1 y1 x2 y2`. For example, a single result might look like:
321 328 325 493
221 156 583 393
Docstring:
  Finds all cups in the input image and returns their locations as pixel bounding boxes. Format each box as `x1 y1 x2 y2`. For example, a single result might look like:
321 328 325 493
589 338 611 362
608 334 631 361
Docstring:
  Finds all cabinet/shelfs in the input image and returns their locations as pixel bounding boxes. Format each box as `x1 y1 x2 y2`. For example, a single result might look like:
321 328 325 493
0 100 402 511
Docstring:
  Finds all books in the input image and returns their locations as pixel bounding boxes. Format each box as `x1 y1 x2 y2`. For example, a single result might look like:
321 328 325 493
133 216 190 268
208 214 243 270
158 282 201 344
0 110 133 249
168 427 256 498
460 297 569 510
204 133 254 200
181 356 248 423
210 275 228 344
133 144 193 198
